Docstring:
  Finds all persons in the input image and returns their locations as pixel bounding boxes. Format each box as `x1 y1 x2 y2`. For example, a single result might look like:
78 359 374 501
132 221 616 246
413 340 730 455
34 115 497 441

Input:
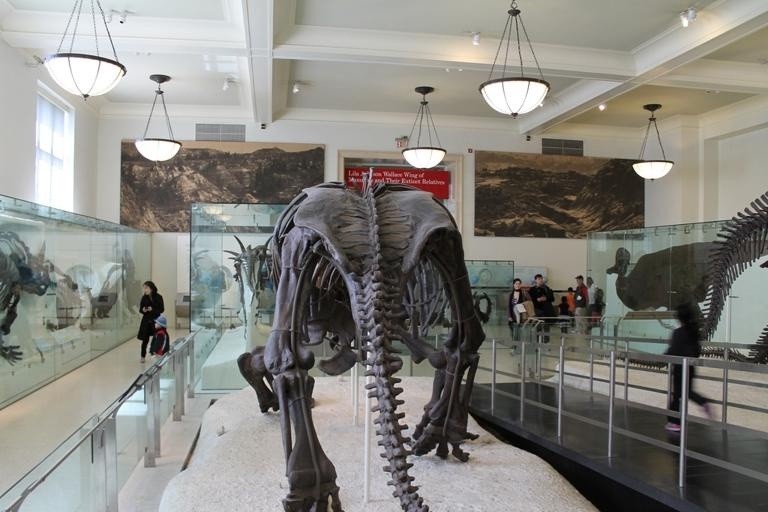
136 280 164 363
149 316 171 356
508 273 603 350
663 302 714 432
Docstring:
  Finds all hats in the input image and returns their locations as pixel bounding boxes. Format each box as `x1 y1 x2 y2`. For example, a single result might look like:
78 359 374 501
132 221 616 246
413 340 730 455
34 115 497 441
154 316 166 327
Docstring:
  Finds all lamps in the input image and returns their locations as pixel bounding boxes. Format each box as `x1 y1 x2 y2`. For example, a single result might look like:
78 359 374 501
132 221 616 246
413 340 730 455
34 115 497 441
471 31 481 45
631 104 675 184
116 6 135 25
477 0 555 119
397 86 450 169
36 0 130 100
675 5 698 27
135 70 184 165
103 7 120 24
291 80 301 96
221 75 234 91
